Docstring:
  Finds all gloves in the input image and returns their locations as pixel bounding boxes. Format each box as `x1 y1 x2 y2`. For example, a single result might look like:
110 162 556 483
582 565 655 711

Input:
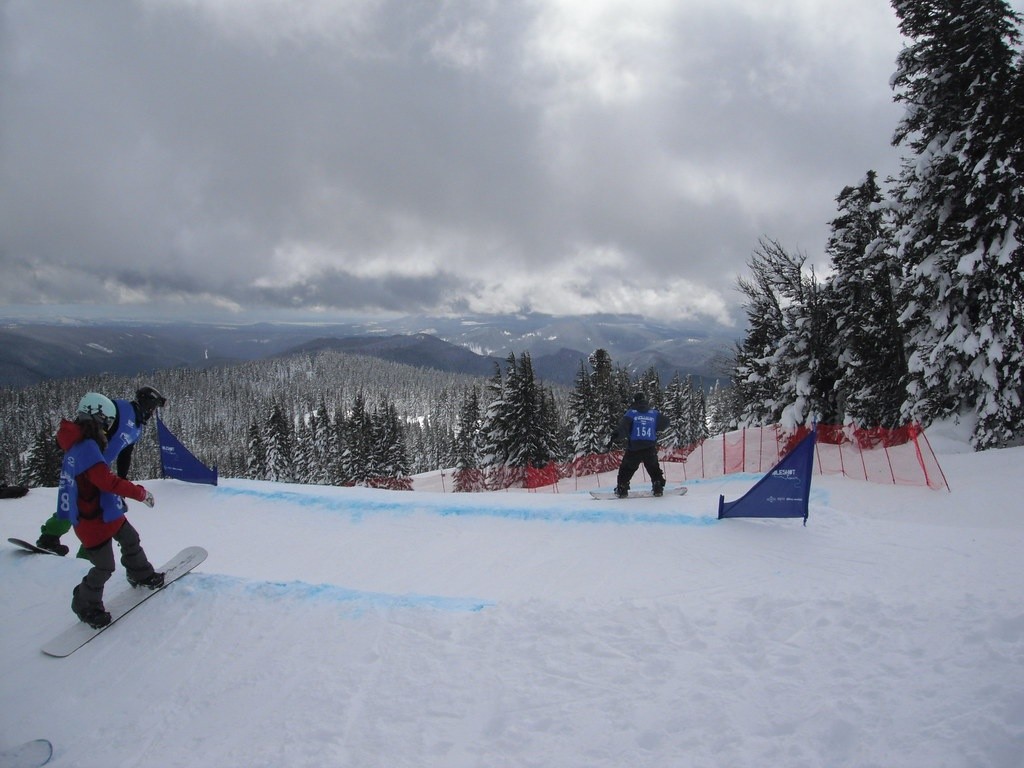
143 491 154 508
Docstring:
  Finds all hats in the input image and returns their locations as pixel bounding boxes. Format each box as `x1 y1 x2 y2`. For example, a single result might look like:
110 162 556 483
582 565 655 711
634 393 645 402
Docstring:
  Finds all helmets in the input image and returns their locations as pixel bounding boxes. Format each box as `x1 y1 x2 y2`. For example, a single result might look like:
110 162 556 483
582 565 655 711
78 393 117 432
133 387 168 415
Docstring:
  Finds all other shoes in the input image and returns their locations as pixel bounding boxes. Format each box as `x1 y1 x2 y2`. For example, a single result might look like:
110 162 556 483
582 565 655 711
654 488 663 496
614 488 628 498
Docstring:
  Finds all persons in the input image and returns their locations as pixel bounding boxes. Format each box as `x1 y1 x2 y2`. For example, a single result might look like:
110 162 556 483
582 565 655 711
611 393 670 499
58 393 165 629
38 385 167 557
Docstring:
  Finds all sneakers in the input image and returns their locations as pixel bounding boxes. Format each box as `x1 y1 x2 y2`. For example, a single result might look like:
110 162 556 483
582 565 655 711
128 572 164 590
77 610 111 630
36 535 69 556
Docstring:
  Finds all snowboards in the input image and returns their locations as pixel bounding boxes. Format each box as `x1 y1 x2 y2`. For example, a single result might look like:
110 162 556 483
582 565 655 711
7 532 47 555
38 543 211 659
587 487 690 500
